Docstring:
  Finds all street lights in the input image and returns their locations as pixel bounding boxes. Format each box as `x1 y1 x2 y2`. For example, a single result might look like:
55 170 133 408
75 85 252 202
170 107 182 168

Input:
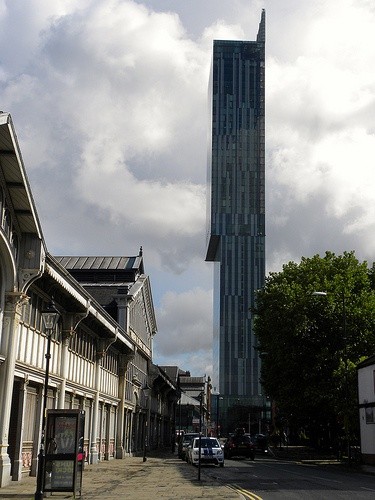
140 380 151 463
196 390 207 481
215 392 220 439
35 295 63 499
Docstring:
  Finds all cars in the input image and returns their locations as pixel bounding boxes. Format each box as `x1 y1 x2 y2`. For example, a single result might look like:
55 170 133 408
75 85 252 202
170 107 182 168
187 437 226 468
174 429 205 460
224 436 255 461
